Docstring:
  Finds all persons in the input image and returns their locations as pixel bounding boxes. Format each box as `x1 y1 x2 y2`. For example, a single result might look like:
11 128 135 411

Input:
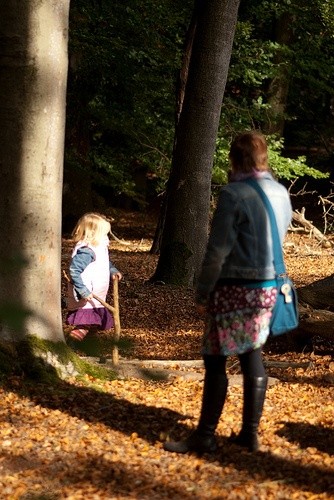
164 133 292 452
65 212 120 345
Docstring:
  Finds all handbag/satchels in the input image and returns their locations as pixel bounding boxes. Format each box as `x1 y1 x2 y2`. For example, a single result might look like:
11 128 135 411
268 277 299 337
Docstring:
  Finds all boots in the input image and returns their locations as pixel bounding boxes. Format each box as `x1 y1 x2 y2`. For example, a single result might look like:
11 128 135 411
66 335 80 352
228 374 268 452
162 373 229 453
